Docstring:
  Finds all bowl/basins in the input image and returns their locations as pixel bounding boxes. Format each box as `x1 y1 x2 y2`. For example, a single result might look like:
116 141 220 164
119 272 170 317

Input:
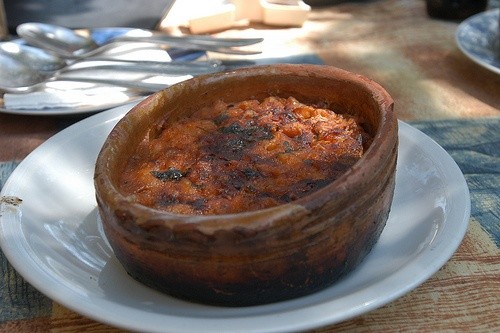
94 64 399 307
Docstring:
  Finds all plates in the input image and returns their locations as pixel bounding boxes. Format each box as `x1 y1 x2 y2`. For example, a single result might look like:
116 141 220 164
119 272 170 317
0 96 472 333
0 28 194 114
454 8 500 74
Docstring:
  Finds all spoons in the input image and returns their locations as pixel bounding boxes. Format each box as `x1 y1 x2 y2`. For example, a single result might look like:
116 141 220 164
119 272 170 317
0 22 265 94
